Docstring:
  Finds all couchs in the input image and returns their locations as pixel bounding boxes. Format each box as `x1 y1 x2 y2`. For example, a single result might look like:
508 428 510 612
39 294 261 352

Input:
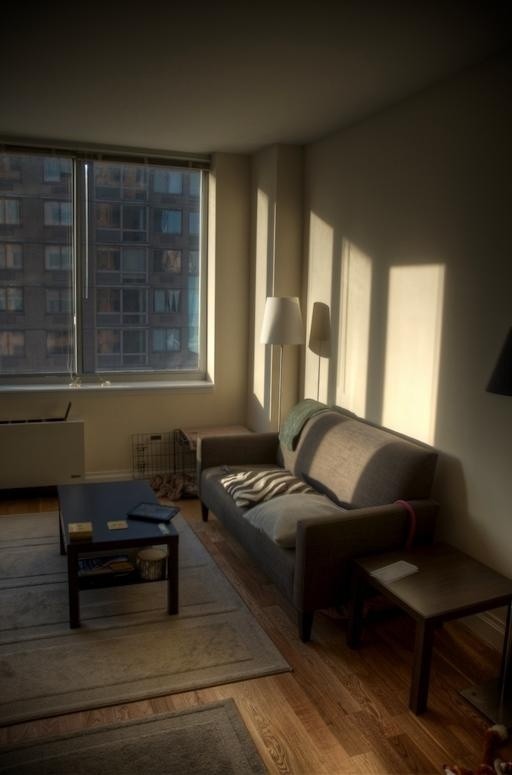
196 400 440 643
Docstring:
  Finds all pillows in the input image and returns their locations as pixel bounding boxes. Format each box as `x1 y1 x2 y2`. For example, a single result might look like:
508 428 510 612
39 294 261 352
217 470 350 547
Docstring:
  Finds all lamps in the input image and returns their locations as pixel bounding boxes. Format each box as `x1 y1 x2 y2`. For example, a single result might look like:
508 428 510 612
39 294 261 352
258 297 305 432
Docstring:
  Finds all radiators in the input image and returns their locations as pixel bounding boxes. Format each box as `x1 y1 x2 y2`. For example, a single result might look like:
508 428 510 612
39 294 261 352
0 418 84 489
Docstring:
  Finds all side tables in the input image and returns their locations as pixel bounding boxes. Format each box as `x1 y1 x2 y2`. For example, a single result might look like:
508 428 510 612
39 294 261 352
346 541 512 714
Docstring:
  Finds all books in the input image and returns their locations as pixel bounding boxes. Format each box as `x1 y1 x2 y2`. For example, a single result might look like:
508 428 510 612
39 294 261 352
79 556 134 577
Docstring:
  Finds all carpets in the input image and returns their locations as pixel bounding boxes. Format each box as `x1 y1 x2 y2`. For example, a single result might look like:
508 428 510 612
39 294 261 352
3 697 270 772
1 508 292 725
